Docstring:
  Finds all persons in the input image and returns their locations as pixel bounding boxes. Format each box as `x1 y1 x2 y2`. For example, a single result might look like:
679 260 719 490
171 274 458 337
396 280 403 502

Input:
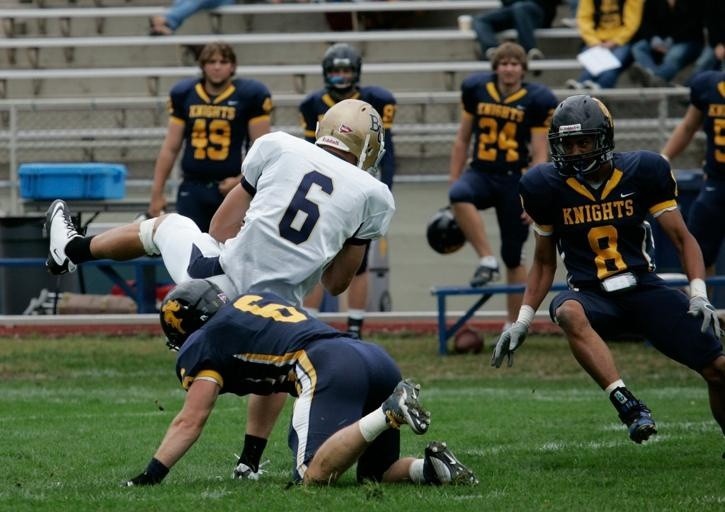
658 18 724 310
121 275 480 488
44 99 395 482
147 39 272 243
489 93 724 464
299 42 397 342
449 41 557 343
472 0 725 89
149 0 221 35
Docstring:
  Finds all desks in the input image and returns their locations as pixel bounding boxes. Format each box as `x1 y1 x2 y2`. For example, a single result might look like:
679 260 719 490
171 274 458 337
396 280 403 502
20 196 177 310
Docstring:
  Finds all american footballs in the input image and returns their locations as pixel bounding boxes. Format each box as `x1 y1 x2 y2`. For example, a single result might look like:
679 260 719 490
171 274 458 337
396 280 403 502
455 329 480 354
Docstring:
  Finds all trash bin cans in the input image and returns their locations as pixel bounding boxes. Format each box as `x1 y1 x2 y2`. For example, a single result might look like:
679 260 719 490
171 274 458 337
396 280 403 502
337 233 393 313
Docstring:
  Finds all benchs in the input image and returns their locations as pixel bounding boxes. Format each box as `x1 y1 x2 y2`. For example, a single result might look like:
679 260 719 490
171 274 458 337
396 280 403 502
0 0 725 180
428 274 724 354
1 255 164 316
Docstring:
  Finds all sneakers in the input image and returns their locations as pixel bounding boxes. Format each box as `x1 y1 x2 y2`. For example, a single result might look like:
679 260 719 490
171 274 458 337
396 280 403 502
384 377 431 435
618 400 657 444
42 199 84 275
149 15 172 35
471 264 501 287
231 464 262 482
424 440 478 486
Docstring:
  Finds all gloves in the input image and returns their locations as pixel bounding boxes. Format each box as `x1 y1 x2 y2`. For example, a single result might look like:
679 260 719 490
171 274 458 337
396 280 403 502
687 295 723 339
491 322 529 367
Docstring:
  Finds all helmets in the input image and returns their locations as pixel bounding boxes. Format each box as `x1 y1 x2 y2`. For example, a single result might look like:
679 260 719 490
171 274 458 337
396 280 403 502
159 278 229 350
427 205 465 254
547 95 614 177
315 98 385 171
323 44 361 87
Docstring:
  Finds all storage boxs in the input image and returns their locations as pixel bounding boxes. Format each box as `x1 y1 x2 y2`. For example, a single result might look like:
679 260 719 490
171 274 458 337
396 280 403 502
17 162 127 200
